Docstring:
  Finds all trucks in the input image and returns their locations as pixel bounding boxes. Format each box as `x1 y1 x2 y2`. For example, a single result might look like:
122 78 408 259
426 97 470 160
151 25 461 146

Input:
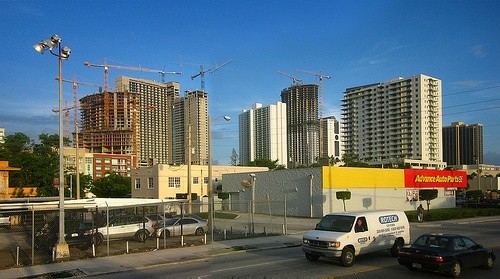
302 209 411 268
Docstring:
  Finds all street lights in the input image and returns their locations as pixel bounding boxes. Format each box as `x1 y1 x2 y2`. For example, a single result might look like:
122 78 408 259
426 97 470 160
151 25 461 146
32 33 72 264
207 113 233 242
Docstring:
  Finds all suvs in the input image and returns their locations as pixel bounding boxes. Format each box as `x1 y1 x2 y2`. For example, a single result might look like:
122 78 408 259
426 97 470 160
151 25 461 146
88 214 156 249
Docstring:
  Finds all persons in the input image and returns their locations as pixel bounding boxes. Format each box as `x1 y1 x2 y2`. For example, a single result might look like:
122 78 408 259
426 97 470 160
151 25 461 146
417 204 424 223
355 219 365 233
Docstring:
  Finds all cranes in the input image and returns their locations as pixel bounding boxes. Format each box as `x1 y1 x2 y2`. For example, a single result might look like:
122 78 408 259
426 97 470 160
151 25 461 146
190 60 233 90
275 70 303 85
62 76 103 134
294 68 333 118
84 56 184 128
51 101 86 140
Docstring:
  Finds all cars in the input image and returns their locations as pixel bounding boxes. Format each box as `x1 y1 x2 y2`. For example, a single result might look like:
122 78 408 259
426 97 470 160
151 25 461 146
48 219 91 250
0 215 12 230
145 213 209 239
396 233 497 277
455 190 487 207
